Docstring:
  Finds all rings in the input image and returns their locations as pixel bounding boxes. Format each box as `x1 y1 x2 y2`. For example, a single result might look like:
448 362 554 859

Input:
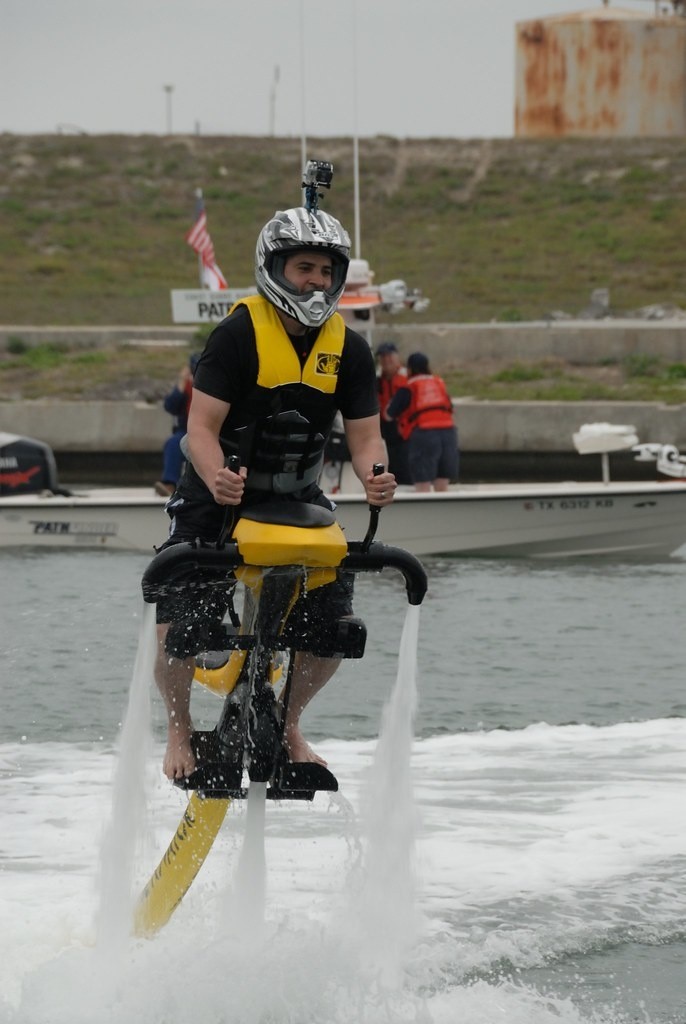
381 491 385 499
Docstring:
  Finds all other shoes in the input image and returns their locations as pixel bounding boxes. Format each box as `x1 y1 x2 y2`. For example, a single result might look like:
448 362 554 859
155 482 175 497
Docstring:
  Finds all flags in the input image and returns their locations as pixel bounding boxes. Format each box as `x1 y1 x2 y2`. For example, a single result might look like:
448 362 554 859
184 195 229 293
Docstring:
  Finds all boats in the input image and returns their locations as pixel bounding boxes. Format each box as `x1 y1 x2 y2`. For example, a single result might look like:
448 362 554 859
0 0 686 561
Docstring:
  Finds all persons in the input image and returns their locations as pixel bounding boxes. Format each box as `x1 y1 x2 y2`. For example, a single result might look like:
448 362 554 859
374 342 458 492
152 206 398 782
154 365 194 496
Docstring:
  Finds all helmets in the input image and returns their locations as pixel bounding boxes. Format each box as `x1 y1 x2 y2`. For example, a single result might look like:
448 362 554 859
254 158 352 328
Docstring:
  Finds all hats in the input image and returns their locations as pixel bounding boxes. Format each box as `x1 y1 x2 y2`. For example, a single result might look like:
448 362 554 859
190 353 201 373
409 351 428 368
375 343 395 356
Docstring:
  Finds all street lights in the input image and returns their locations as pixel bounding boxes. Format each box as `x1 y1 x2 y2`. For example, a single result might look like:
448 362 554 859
163 84 173 135
